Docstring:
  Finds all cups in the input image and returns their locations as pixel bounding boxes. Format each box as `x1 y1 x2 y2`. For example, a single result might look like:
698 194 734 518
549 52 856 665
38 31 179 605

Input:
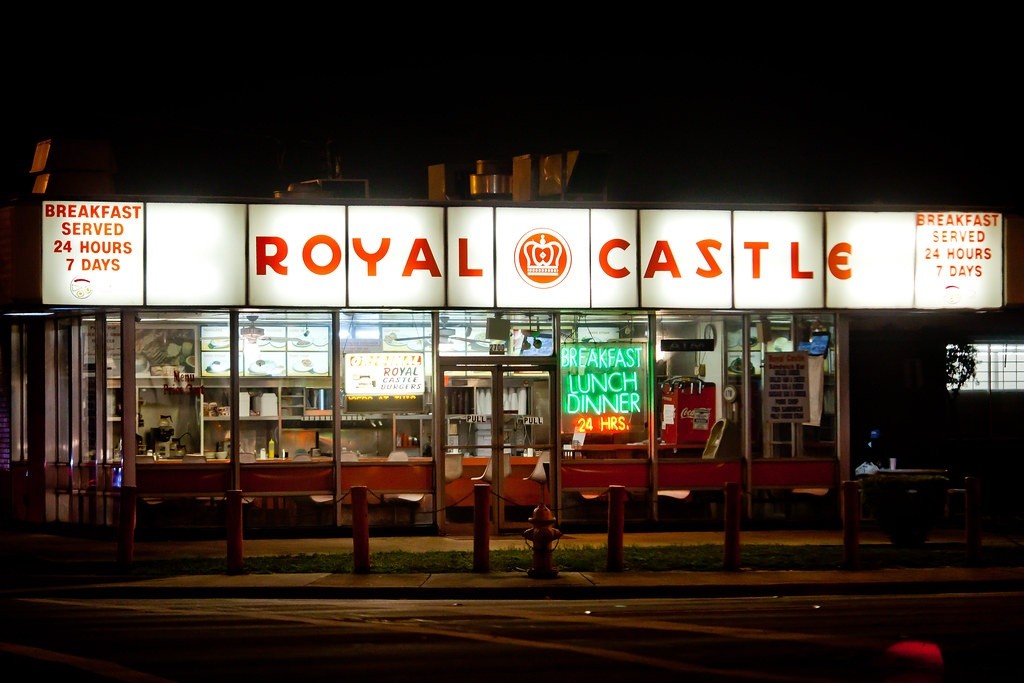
450 389 471 414
503 388 526 415
477 389 491 414
269 438 274 459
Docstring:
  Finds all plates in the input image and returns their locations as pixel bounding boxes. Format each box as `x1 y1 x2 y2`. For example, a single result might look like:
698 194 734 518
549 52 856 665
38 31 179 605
208 335 328 373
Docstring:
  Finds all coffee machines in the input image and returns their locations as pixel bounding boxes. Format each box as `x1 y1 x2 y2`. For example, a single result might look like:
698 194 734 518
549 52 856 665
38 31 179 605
151 427 174 459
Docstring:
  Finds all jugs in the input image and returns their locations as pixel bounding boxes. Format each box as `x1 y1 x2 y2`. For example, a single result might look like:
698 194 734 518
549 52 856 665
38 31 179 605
159 415 173 426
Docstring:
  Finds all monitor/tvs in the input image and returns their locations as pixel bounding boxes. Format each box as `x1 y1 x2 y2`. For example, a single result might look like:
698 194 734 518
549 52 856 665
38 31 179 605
798 331 831 359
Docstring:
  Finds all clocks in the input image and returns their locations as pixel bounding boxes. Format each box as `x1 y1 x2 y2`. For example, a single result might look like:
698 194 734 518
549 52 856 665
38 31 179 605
703 323 716 339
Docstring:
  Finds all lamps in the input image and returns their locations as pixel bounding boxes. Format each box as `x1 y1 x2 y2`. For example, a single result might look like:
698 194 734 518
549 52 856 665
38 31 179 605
240 315 264 343
303 323 309 336
465 317 471 337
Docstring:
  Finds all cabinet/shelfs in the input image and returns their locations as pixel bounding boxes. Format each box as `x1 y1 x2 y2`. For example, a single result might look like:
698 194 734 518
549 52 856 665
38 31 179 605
199 385 283 460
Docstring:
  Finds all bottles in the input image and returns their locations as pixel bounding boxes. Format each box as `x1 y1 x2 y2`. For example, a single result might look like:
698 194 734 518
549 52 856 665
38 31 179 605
863 430 885 470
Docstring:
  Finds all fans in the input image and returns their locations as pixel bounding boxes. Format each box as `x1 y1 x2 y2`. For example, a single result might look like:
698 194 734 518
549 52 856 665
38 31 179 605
394 317 491 343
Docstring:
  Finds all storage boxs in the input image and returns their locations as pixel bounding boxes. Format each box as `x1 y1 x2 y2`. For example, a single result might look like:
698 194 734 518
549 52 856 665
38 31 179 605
227 390 250 417
252 392 277 415
447 421 491 456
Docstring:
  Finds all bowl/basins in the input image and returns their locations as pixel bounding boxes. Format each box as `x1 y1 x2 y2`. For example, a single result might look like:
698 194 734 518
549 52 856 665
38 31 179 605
186 356 195 373
216 452 228 459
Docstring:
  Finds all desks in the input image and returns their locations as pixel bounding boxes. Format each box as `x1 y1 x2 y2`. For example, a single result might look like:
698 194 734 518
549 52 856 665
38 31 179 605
874 469 948 545
